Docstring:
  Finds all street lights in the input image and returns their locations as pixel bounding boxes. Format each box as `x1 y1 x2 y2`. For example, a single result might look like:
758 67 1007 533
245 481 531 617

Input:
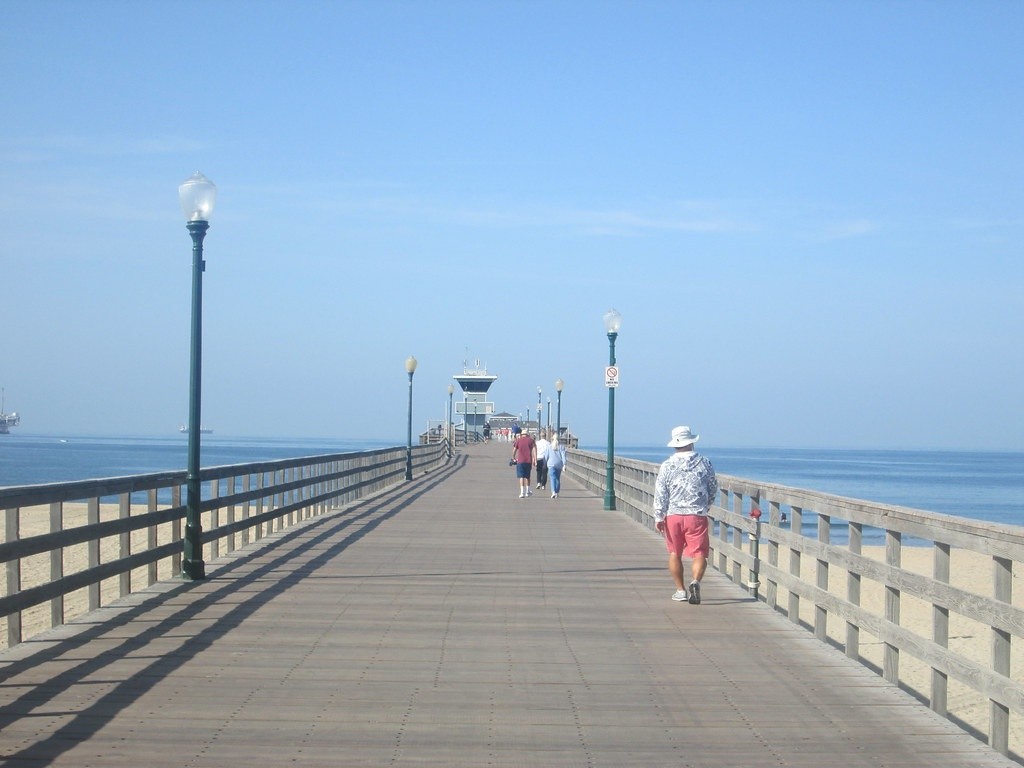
536 386 543 440
401 356 418 480
447 383 456 461
601 309 621 511
556 379 564 446
547 396 552 429
471 399 478 444
174 171 213 583
464 390 471 449
525 404 532 438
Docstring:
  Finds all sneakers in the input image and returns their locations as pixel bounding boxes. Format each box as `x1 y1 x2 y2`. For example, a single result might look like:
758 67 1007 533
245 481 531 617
688 580 700 604
672 591 688 602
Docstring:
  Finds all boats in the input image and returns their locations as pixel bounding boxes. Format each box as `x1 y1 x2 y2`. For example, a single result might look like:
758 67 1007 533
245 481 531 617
0 385 21 435
180 424 213 436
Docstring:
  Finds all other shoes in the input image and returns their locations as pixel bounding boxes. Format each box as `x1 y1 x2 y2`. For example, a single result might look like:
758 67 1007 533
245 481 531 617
519 493 525 498
536 483 540 489
525 491 532 497
540 486 545 490
551 493 556 498
555 493 559 498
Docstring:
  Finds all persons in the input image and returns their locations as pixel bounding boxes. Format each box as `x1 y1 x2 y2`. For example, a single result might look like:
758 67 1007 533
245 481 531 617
512 422 519 438
511 430 537 498
544 433 567 498
497 427 511 442
653 426 716 605
533 430 551 489
482 421 490 445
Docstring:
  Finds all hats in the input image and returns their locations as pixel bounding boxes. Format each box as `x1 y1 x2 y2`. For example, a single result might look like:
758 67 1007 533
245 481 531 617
667 425 699 448
521 429 528 434
